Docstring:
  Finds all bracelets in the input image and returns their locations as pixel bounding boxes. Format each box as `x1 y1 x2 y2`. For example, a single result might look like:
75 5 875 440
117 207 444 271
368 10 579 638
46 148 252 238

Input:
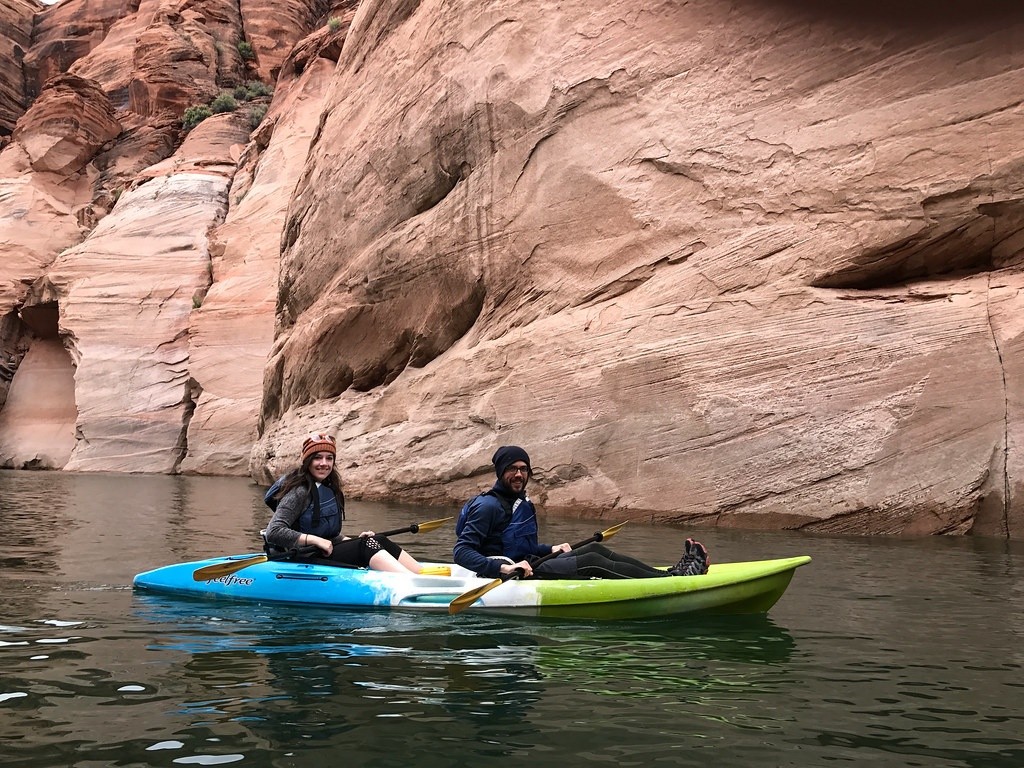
305 534 309 546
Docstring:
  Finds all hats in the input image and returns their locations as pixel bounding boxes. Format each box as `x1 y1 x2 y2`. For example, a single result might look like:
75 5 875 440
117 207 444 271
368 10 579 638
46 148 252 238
302 434 336 464
492 446 530 479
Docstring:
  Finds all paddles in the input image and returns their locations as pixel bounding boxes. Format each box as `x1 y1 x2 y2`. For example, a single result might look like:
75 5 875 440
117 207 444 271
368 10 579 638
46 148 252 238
192 515 455 582
448 519 627 615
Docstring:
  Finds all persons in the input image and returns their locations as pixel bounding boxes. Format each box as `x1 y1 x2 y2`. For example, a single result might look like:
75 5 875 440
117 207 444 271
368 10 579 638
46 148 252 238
453 446 710 580
266 434 422 574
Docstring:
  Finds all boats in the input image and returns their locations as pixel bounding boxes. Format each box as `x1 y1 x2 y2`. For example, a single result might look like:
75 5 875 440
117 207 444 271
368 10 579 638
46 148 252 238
134 554 812 624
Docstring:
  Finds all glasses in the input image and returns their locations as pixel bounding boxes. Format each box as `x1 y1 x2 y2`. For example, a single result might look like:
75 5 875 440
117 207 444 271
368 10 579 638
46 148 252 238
507 465 529 473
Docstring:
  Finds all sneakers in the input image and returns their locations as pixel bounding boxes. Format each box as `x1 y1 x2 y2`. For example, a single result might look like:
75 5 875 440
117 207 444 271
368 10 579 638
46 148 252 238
667 538 695 576
669 542 711 576
